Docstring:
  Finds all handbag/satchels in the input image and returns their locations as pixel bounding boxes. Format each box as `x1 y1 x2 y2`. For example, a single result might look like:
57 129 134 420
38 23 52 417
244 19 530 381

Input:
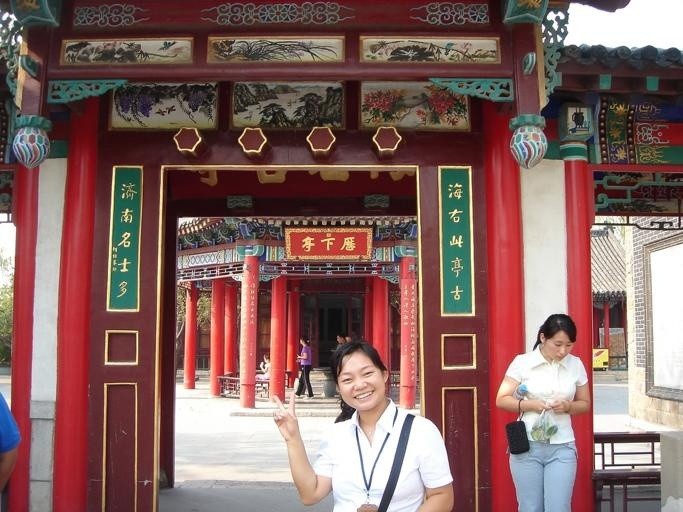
506 399 530 454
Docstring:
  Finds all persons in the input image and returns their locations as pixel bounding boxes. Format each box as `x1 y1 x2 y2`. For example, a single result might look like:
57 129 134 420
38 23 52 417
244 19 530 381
255 334 456 512
256 353 271 381
292 335 314 400
335 334 347 350
0 391 21 494
495 314 591 512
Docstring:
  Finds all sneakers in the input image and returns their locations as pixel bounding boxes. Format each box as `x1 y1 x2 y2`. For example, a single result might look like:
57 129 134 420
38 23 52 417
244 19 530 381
289 394 299 399
303 396 314 399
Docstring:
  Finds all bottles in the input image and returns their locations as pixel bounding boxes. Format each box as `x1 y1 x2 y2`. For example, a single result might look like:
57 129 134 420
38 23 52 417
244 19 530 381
513 385 527 400
